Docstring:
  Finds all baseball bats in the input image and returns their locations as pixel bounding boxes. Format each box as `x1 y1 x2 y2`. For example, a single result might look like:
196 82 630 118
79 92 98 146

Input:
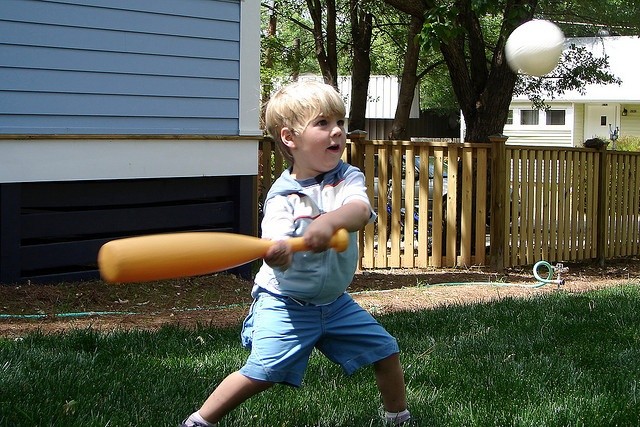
98 228 349 282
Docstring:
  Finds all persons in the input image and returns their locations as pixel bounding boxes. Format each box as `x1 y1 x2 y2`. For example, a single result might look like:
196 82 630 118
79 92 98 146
181 73 412 427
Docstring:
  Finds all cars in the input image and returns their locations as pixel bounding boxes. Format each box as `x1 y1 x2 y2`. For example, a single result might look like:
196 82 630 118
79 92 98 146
364 151 523 235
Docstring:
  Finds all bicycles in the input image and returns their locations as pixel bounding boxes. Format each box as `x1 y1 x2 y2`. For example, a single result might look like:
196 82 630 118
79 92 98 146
365 180 442 255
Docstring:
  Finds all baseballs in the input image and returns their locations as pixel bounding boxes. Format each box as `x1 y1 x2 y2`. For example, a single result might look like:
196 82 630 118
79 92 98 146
505 18 565 76
